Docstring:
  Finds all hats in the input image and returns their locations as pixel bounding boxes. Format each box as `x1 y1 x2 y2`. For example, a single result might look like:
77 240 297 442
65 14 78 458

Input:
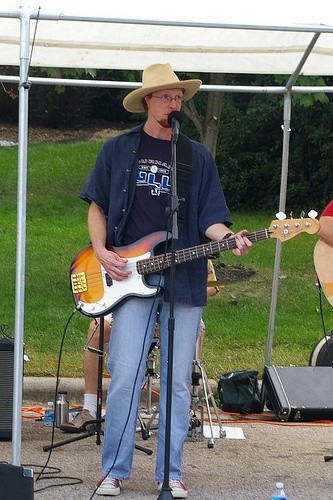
123 63 202 113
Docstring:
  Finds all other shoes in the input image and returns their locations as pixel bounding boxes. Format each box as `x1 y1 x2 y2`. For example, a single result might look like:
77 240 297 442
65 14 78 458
157 479 188 498
95 477 122 496
60 410 95 433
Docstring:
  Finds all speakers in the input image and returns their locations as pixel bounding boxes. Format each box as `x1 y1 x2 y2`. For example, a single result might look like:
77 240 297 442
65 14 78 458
262 365 333 422
0 339 25 442
0 464 34 500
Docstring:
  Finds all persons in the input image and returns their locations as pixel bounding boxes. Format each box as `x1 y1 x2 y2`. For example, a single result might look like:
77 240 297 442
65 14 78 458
87 63 252 498
59 258 219 433
316 199 333 246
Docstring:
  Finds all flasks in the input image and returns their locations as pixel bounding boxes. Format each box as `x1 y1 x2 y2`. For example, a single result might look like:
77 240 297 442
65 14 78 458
56 391 69 428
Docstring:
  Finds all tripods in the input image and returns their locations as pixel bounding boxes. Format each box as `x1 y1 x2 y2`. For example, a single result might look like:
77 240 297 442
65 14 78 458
141 336 227 449
42 316 153 455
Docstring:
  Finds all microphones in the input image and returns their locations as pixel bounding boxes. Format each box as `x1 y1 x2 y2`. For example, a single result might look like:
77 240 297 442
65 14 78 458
168 111 184 143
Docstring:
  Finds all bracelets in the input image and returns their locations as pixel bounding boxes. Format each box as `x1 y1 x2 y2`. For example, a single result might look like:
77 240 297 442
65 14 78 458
222 232 234 240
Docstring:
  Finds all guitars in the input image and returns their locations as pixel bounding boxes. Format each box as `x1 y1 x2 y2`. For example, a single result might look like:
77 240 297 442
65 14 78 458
313 239 333 308
70 210 321 317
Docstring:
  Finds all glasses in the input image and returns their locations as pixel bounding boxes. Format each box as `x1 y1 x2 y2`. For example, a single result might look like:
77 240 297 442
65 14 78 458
150 94 182 103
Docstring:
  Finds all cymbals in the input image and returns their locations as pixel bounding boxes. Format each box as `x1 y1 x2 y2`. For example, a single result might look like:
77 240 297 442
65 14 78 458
207 280 224 287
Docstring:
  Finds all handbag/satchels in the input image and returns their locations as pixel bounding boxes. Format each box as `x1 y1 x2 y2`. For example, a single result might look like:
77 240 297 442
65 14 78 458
218 369 262 414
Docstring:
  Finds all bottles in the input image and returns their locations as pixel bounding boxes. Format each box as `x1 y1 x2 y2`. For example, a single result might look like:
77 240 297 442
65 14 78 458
272 483 288 500
44 402 54 426
